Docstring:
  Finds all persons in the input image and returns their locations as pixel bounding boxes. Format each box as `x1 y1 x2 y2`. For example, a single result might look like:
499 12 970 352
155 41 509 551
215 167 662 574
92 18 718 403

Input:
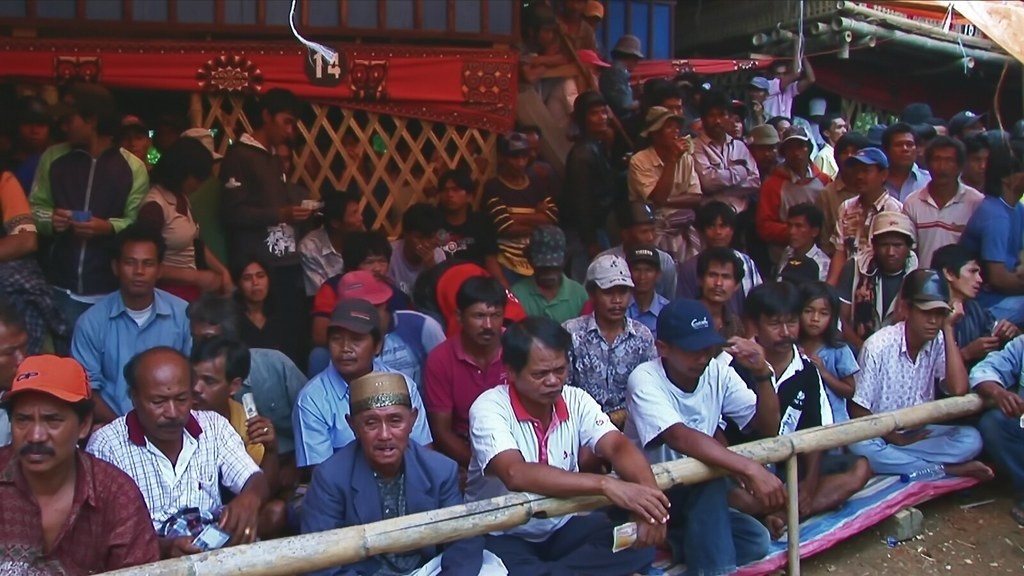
0 0 1024 576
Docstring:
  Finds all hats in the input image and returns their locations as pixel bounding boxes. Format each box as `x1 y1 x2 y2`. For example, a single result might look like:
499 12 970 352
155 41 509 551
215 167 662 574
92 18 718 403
747 124 781 145
584 255 636 290
846 147 889 169
578 35 645 68
640 106 686 138
180 128 225 161
873 211 916 242
866 124 888 145
328 298 379 335
50 82 117 117
782 126 809 143
2 354 93 403
527 223 568 269
627 245 661 267
349 372 411 414
913 300 954 312
948 111 984 136
656 298 727 353
749 77 770 90
118 114 147 130
338 270 394 305
583 0 604 20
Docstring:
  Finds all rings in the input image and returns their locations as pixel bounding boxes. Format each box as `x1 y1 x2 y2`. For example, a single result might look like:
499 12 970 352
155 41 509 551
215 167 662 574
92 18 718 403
244 527 251 535
263 427 269 434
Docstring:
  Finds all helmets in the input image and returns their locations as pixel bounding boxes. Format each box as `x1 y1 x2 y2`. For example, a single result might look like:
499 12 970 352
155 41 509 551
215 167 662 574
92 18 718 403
524 4 558 29
13 96 53 125
623 201 657 224
901 268 950 301
